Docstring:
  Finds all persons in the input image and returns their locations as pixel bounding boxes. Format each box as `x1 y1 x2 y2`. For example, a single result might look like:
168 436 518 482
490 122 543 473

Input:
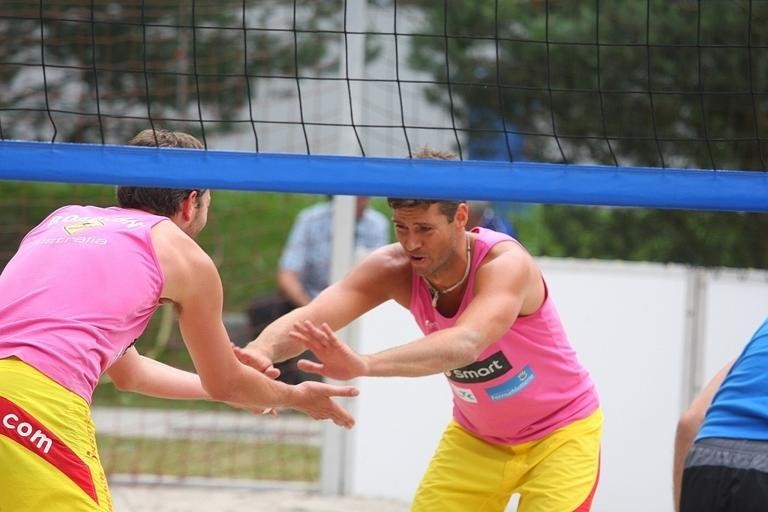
453 38 533 247
664 318 768 511
272 182 388 405
0 129 360 511
221 142 605 512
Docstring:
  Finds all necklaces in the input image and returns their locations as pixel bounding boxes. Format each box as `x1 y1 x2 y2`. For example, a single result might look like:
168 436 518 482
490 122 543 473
420 239 483 311
